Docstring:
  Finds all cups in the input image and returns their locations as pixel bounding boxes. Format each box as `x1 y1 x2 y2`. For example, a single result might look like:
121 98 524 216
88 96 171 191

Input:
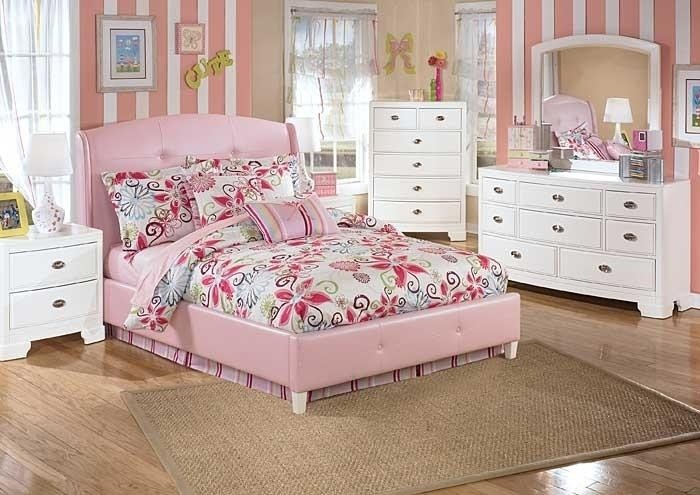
408 87 424 101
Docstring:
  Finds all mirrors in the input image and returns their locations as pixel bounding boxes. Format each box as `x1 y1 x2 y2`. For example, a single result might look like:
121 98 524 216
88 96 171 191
526 36 665 164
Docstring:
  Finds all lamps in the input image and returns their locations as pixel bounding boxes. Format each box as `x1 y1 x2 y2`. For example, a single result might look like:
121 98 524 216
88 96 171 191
601 92 636 146
20 130 80 237
284 115 322 175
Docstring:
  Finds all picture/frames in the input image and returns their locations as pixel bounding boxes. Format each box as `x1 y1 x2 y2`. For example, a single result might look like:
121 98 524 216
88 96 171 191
667 61 699 152
92 12 155 92
173 21 208 58
0 188 33 238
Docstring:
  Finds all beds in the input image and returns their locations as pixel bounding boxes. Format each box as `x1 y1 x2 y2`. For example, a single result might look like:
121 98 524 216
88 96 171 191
70 112 524 417
539 88 628 159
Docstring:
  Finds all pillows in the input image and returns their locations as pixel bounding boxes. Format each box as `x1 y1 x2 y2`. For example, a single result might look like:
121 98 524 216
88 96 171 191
97 152 344 255
556 124 629 159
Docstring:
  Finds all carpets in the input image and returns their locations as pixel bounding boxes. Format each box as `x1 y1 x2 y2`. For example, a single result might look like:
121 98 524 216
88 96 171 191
115 331 699 495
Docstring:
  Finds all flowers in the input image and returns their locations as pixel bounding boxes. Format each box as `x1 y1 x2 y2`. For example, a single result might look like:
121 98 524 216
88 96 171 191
428 49 447 67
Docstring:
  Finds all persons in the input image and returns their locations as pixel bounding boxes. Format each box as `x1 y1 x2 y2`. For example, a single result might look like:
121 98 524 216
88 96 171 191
695 93 700 126
0 203 21 231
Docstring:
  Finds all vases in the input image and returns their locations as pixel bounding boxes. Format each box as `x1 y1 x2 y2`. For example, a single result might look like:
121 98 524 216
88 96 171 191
435 68 443 101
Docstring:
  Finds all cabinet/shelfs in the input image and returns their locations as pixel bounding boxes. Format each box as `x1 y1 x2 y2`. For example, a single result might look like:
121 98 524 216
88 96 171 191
475 159 697 325
366 96 472 242
311 169 339 196
506 125 553 175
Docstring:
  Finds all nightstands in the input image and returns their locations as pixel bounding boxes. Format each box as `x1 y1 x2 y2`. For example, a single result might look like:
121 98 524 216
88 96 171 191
316 195 353 214
0 218 108 367
